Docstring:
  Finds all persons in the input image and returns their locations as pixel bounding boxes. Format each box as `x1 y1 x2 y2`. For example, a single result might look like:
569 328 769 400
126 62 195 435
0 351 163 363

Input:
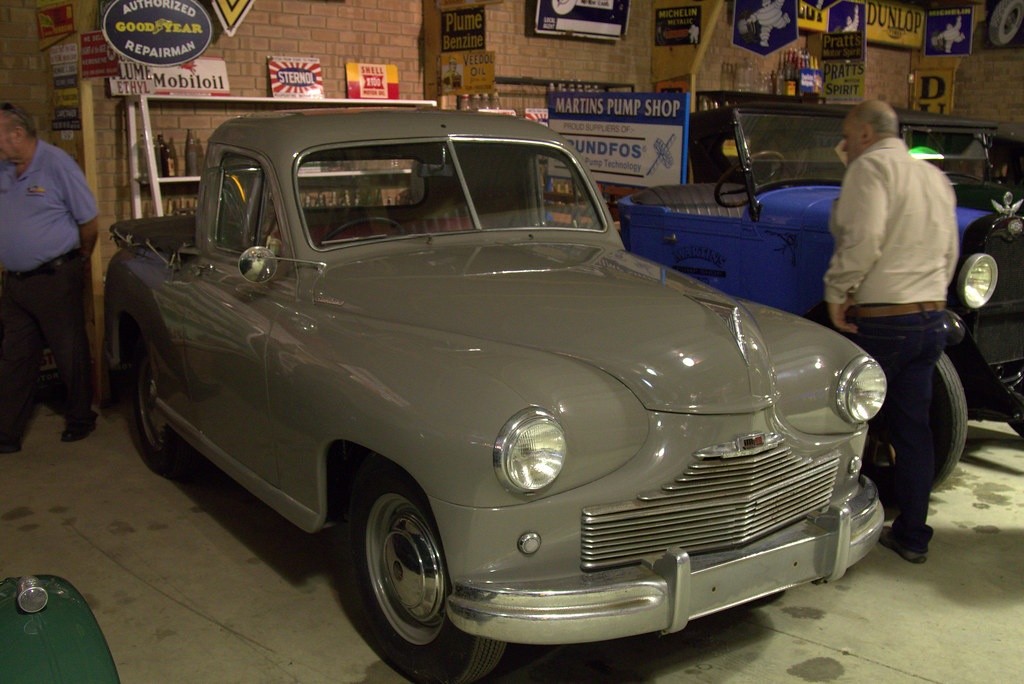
0 102 98 454
822 100 959 564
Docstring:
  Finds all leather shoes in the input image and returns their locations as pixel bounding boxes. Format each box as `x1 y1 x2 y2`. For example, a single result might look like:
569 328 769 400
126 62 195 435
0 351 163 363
0 441 20 453
62 422 96 442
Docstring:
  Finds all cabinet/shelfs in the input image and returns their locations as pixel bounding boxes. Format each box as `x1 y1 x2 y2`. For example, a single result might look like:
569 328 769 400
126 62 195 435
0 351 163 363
123 95 438 218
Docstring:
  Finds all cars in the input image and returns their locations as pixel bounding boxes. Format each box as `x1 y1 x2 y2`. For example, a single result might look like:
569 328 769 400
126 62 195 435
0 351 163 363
102 107 882 684
531 88 1024 491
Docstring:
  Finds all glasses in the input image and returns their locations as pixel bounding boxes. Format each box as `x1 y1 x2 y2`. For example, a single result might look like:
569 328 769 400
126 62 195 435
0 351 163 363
2 103 14 110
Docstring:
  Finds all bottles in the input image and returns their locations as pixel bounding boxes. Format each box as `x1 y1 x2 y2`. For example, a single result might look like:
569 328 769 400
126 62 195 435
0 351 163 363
461 93 472 111
699 95 737 111
473 93 482 112
139 130 200 177
482 93 491 110
550 178 578 205
302 191 400 208
146 197 198 217
492 92 502 109
722 48 822 95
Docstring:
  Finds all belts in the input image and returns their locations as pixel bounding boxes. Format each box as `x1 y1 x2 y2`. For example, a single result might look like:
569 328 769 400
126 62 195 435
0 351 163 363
848 301 945 317
8 249 80 280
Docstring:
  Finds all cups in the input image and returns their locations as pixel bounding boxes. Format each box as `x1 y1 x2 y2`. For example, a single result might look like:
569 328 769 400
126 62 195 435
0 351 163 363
16 576 47 613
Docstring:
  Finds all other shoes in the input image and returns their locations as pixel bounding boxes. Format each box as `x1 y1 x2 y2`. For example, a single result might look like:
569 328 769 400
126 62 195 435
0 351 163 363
879 526 928 563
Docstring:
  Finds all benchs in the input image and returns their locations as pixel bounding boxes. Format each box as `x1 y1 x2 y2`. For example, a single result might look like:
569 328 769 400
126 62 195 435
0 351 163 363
631 183 750 218
308 217 474 244
